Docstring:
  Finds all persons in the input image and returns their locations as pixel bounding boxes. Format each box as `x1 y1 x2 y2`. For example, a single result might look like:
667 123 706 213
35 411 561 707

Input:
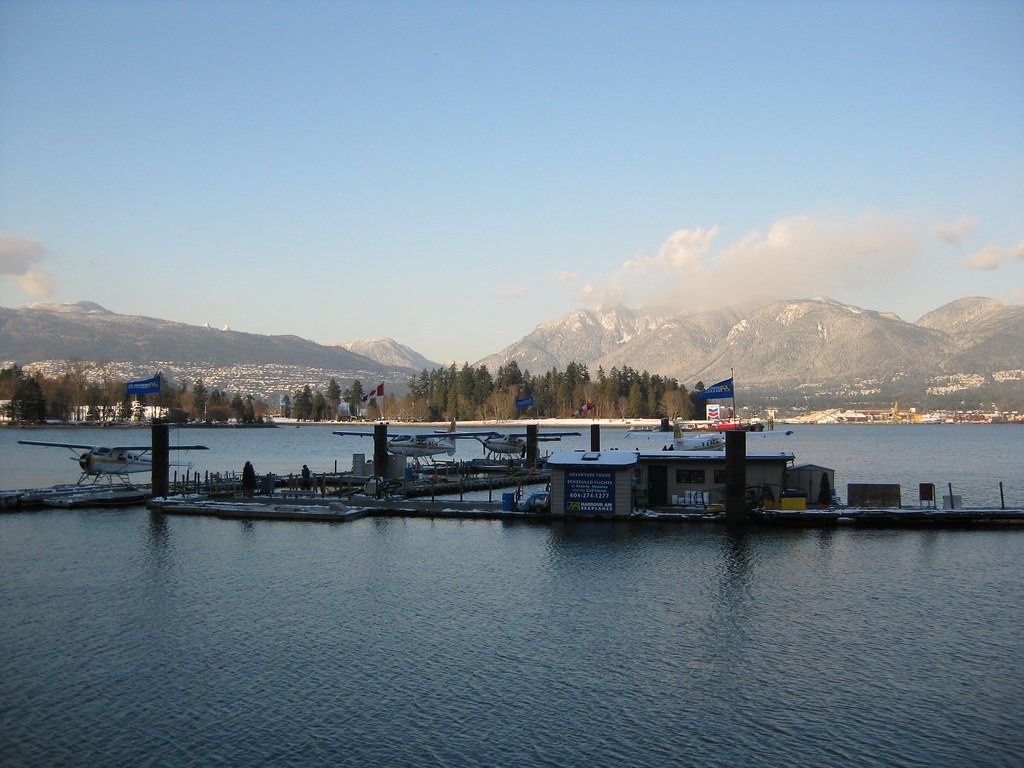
242 461 257 497
818 471 831 508
301 465 310 491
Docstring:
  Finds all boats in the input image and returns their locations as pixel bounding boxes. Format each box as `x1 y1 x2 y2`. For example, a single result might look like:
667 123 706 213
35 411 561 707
671 421 725 451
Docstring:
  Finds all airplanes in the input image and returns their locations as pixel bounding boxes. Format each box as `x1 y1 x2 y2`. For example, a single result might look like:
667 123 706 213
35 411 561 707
18 439 210 487
432 428 581 460
331 416 503 470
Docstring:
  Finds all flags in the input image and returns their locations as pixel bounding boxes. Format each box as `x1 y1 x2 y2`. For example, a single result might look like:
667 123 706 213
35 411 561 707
125 376 161 395
516 396 533 408
696 378 734 400
362 384 384 401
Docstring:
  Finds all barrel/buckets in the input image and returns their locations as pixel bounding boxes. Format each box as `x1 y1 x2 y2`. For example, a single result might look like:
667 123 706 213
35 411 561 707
671 491 709 506
501 492 514 512
